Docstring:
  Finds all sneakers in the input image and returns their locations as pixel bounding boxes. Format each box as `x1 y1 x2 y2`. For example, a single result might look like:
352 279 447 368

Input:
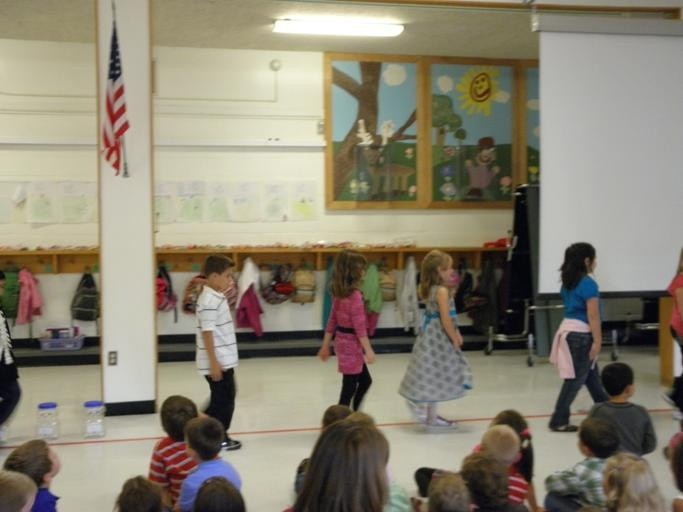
218 437 241 453
661 388 683 423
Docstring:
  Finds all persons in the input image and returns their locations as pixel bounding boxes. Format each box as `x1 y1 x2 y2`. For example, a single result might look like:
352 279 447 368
543 362 682 510
0 269 21 445
398 249 474 428
662 246 683 409
115 395 245 512
1 439 60 510
548 241 609 433
193 255 242 451
289 405 539 511
316 252 377 415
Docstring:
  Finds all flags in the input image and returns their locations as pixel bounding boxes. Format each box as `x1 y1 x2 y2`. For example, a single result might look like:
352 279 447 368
99 18 131 178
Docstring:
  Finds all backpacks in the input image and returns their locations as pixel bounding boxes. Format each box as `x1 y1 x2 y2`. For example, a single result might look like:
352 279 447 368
181 275 208 315
155 267 177 312
0 271 22 319
378 263 397 302
454 272 490 314
291 268 318 303
262 264 293 305
219 276 239 310
71 274 99 322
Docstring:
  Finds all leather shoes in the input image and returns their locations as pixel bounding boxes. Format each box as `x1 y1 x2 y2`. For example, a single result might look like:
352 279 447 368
551 422 578 433
412 408 457 430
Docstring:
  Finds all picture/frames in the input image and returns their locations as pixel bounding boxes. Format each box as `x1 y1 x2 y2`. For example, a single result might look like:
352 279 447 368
325 50 428 214
518 58 539 188
427 55 518 210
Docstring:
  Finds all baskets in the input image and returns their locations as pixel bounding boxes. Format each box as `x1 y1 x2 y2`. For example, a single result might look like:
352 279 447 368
38 333 86 352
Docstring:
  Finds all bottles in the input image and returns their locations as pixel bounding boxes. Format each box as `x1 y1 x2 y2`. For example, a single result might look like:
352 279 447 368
34 401 58 440
79 399 106 440
